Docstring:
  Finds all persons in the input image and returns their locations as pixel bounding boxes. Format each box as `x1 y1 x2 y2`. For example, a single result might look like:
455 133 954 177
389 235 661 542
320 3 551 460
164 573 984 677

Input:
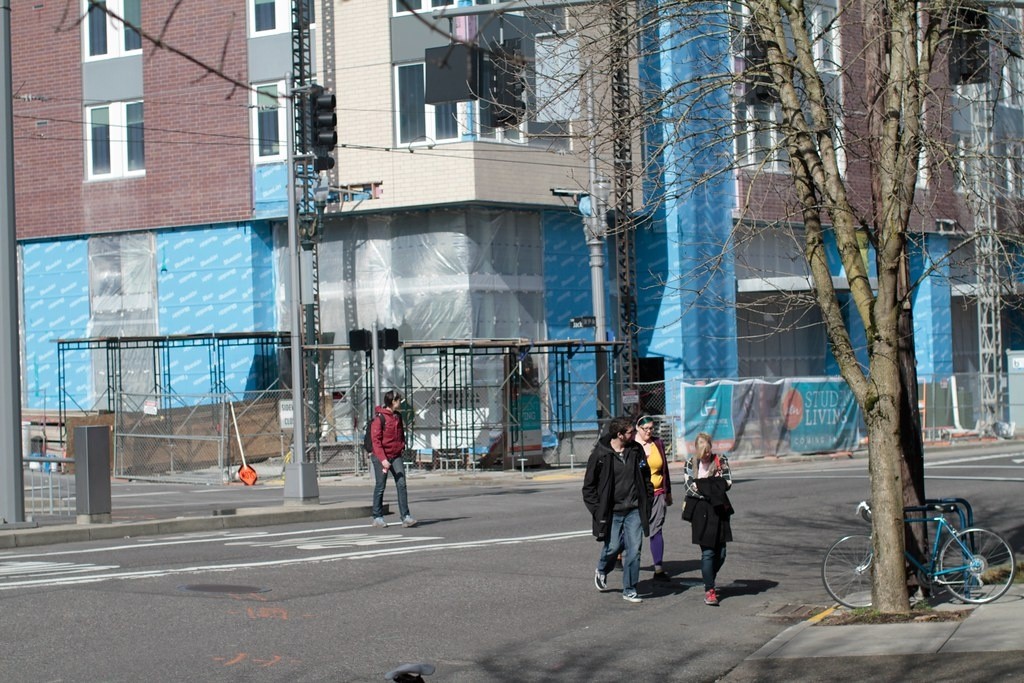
365 390 418 528
681 433 735 605
581 416 655 602
615 414 673 582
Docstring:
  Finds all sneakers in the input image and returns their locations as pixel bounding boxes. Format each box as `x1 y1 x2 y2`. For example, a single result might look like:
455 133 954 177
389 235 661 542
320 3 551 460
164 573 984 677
373 516 387 528
704 588 718 604
402 515 418 528
623 592 643 603
653 570 671 582
614 558 624 571
595 568 608 591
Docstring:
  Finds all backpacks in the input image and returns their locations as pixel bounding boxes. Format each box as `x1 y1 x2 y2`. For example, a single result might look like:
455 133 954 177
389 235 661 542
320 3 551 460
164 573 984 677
363 411 403 453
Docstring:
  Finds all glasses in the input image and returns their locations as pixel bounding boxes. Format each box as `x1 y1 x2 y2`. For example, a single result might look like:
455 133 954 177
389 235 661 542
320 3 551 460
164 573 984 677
640 426 654 432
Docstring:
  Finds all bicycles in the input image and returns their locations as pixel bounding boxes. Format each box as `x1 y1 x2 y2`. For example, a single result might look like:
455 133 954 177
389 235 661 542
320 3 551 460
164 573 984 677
821 501 1016 610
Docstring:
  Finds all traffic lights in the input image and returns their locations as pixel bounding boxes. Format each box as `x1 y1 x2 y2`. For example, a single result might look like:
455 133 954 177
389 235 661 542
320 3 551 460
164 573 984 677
349 328 372 351
379 330 400 349
310 85 338 150
489 38 527 124
